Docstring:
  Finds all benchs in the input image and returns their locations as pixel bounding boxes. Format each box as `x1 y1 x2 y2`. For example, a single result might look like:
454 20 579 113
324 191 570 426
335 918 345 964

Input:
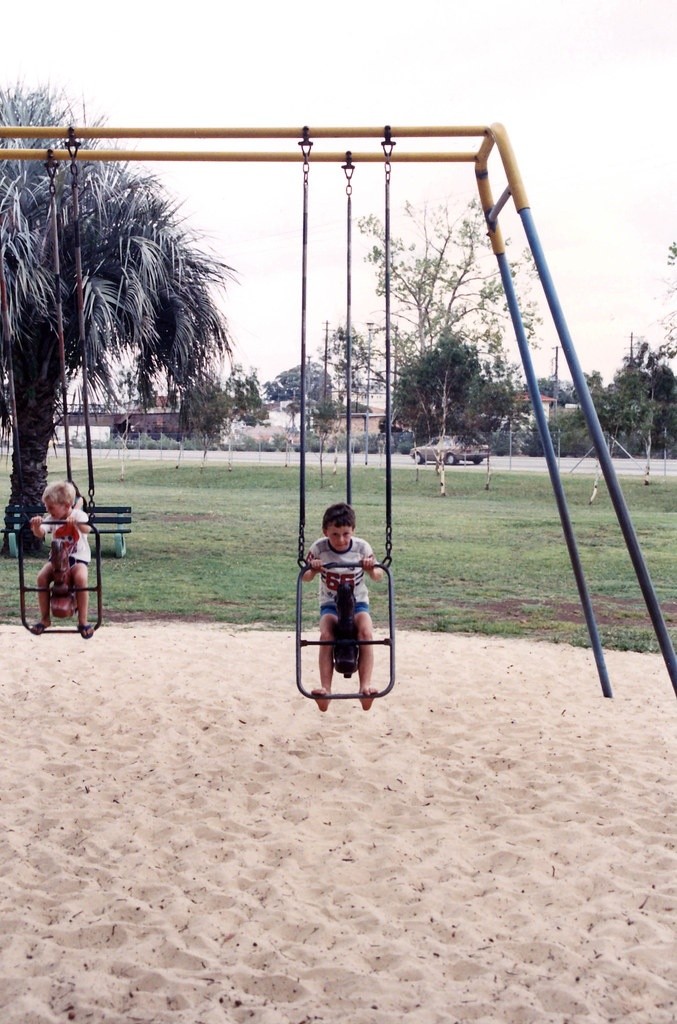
0 505 132 558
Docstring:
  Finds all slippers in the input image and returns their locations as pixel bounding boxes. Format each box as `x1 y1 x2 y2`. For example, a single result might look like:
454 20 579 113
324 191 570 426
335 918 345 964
31 620 51 635
77 625 94 639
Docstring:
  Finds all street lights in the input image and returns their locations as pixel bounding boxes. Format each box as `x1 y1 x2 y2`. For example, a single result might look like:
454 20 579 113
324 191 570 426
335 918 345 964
307 354 311 430
364 322 374 466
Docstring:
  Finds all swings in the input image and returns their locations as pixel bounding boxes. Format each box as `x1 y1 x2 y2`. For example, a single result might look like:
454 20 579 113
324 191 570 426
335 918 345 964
294 123 400 700
0 125 105 634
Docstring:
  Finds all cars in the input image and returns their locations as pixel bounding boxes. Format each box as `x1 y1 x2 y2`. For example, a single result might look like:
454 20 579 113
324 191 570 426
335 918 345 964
409 435 491 465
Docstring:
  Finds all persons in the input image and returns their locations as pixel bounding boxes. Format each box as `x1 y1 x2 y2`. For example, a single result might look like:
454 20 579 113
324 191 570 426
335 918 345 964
30 480 93 639
303 503 383 710
